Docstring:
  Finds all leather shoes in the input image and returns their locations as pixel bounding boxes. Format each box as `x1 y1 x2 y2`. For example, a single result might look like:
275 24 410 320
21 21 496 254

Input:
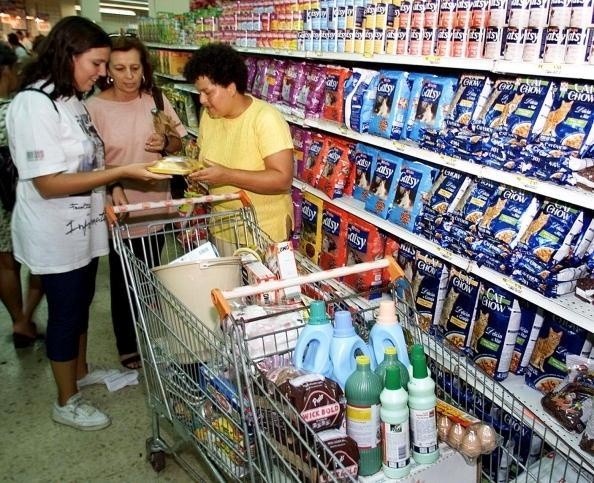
12 322 45 349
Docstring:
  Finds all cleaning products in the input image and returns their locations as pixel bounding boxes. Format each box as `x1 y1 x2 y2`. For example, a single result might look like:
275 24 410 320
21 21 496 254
294 299 440 479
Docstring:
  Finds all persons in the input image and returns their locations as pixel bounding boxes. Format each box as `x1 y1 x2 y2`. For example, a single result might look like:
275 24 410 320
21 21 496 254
0 28 47 98
294 88 434 264
0 41 46 351
180 40 294 273
2 12 178 434
80 29 188 369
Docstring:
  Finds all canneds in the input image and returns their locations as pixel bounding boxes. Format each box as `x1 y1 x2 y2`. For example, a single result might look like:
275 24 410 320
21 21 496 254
194 1 593 69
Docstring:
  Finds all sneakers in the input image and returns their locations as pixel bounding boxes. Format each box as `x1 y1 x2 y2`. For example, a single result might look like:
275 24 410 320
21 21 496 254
76 362 120 387
50 391 110 431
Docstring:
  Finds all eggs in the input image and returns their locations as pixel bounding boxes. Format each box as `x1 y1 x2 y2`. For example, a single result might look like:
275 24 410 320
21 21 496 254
436 410 498 459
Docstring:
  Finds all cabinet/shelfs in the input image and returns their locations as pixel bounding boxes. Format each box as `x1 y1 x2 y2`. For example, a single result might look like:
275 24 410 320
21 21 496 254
140 36 594 483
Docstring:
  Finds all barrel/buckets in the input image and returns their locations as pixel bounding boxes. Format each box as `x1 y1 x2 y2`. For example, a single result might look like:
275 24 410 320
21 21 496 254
150 256 241 364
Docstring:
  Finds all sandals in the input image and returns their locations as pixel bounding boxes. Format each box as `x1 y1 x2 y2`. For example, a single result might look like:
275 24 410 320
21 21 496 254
119 352 142 369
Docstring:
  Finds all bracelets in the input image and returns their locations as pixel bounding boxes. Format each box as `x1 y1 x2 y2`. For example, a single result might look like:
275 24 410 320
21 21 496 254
160 133 170 156
106 180 122 194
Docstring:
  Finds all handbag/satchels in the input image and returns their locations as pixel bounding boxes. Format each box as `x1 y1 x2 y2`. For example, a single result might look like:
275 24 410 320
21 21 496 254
151 86 188 199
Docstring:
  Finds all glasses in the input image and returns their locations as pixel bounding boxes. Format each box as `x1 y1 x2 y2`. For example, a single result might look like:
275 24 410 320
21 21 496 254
109 32 135 38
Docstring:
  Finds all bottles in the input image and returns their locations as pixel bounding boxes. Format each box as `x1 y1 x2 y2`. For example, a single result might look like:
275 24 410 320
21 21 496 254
406 352 440 466
342 353 382 478
378 363 413 480
406 342 432 380
374 345 411 394
